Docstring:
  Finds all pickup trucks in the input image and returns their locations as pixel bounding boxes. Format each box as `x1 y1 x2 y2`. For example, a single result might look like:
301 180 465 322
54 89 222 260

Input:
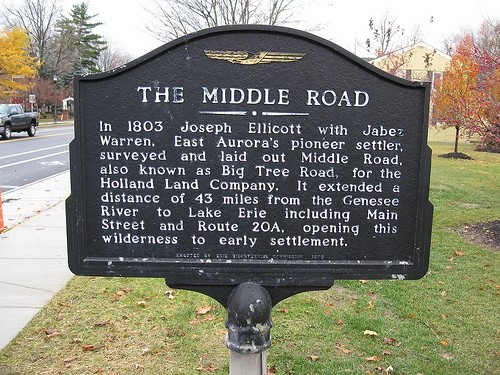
0 103 39 139
